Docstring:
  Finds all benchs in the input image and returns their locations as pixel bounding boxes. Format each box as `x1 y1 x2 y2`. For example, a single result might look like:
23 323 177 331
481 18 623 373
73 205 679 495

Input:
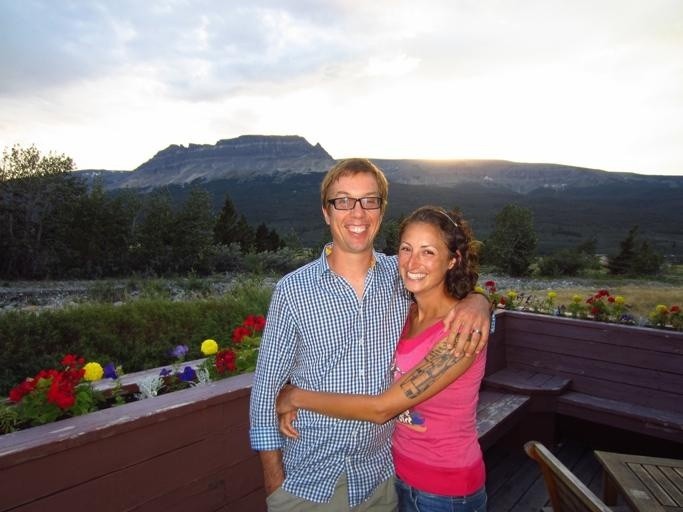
477 309 683 472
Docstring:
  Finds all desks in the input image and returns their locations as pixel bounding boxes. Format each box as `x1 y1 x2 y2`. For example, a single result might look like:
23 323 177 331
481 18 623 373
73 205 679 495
594 450 683 512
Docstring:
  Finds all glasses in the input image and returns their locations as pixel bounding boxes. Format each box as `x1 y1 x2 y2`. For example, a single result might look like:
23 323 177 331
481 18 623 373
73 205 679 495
327 197 383 210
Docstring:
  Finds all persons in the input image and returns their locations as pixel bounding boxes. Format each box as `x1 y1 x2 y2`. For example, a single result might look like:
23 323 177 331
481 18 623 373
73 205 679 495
249 157 497 510
274 202 491 511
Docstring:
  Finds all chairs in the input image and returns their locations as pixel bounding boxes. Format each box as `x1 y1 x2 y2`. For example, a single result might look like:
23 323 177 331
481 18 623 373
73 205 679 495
523 440 613 512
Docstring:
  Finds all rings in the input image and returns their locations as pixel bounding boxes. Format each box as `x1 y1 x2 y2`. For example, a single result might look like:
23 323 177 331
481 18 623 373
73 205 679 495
472 328 482 335
